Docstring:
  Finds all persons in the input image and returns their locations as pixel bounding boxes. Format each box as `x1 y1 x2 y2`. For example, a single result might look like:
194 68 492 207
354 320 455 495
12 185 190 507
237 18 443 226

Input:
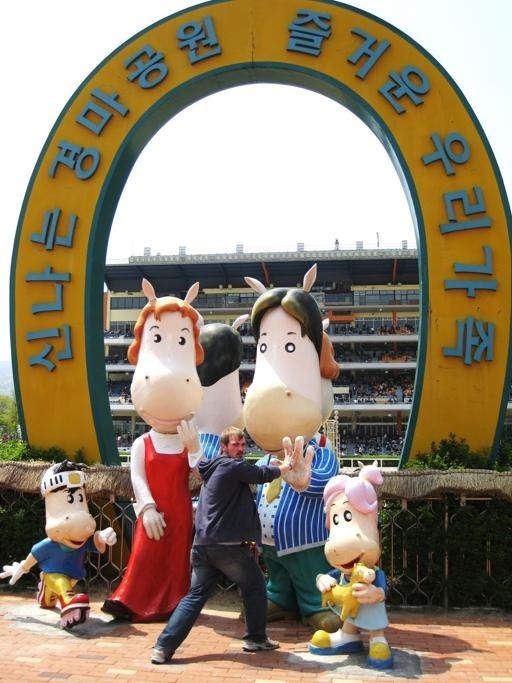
151 427 298 665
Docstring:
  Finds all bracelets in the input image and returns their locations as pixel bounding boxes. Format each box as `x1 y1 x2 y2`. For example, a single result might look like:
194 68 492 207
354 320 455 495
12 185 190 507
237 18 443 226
283 462 293 471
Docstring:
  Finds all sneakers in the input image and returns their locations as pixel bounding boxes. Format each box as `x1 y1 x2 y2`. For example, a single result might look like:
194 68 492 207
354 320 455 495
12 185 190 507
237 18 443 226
242 639 279 654
150 649 173 664
